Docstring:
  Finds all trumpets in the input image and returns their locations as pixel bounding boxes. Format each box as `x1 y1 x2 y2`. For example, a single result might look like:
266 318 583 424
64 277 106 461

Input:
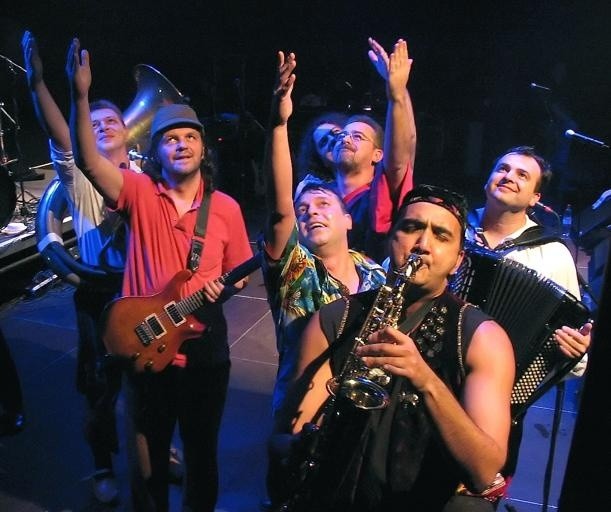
328 254 423 412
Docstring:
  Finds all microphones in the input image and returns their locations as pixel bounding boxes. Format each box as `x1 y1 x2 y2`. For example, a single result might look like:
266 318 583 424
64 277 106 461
530 83 550 92
535 201 557 218
565 129 603 152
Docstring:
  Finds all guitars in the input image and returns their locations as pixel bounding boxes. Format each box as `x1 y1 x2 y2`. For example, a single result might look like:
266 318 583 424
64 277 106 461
98 251 261 383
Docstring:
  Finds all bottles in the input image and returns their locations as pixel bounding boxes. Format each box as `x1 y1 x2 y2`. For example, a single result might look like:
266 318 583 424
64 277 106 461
562 204 573 241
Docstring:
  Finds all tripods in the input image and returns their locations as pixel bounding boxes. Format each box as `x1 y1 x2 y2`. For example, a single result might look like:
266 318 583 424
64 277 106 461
3 57 41 215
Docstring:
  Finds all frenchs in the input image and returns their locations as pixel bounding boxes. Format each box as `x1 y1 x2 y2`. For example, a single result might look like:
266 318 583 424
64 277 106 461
35 64 186 291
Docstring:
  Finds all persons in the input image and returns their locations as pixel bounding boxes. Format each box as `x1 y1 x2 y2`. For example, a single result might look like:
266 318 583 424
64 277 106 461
21 29 186 503
327 38 416 267
295 39 392 185
64 37 256 511
2 163 28 439
445 144 593 511
274 189 517 512
260 48 385 509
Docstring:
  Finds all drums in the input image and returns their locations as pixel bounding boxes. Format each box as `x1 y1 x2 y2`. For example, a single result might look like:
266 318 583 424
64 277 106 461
0 163 17 231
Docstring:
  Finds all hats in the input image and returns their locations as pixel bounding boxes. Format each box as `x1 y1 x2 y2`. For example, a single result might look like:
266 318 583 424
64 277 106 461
151 103 205 139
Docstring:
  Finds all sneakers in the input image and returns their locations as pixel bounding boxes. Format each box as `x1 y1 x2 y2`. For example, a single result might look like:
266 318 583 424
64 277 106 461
88 469 120 504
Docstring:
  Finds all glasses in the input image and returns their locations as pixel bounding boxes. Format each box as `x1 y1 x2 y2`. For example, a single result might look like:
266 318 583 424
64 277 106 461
336 130 379 148
